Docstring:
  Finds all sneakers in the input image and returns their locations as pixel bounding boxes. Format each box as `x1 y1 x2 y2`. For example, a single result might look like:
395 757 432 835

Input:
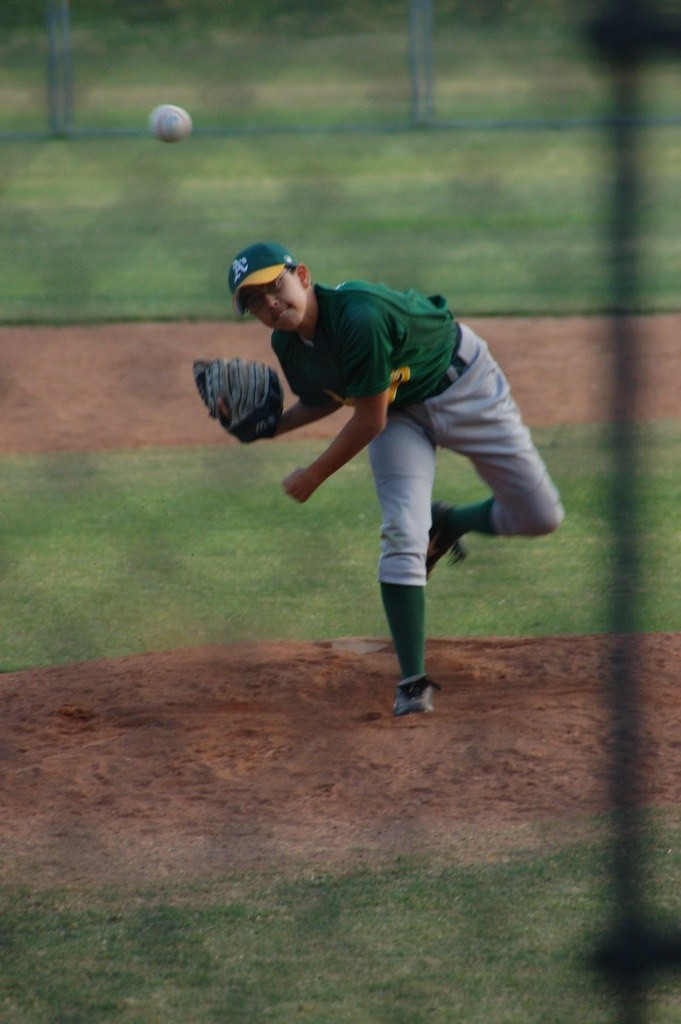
392 673 441 716
425 502 468 581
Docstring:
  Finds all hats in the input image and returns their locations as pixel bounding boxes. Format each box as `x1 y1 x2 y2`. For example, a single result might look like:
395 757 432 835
228 242 297 318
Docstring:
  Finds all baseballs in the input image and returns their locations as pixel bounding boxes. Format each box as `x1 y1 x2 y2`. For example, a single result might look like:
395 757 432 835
147 103 193 142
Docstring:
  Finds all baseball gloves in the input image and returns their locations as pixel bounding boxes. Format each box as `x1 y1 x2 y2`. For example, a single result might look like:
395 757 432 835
193 358 284 444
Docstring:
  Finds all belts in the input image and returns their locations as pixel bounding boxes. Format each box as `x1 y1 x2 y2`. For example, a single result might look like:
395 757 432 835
423 355 468 401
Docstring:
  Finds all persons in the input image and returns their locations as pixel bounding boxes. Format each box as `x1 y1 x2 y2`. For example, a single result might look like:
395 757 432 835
195 241 567 717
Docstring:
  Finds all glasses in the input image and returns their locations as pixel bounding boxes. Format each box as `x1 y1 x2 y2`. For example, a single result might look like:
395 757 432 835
243 265 293 313
